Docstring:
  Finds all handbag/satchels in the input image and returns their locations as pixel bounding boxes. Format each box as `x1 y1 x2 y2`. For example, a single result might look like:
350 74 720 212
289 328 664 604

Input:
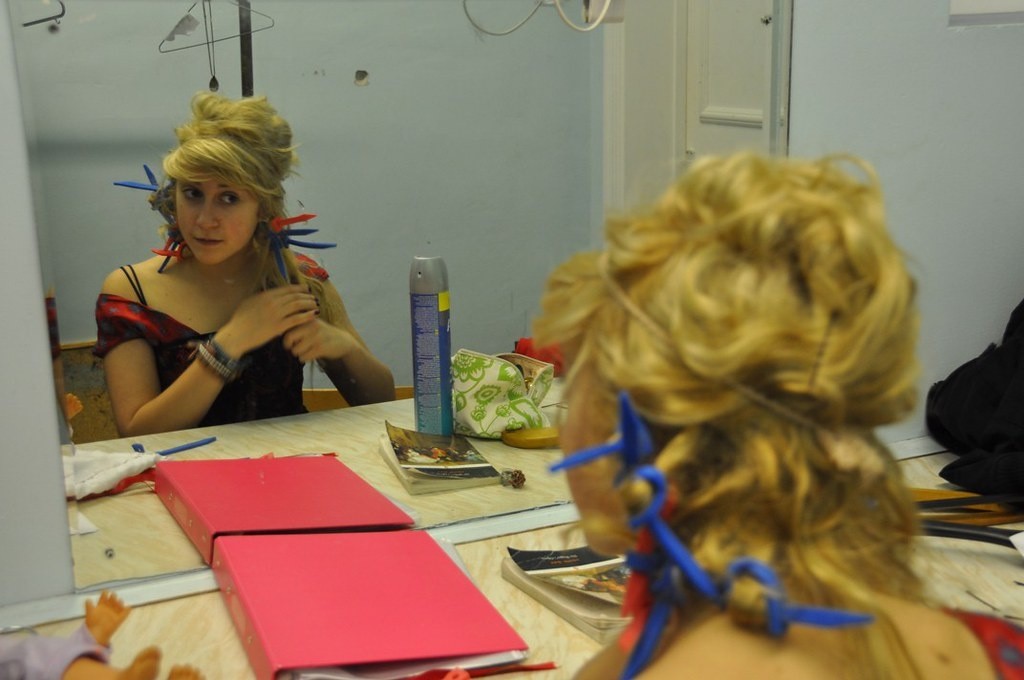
447 345 554 437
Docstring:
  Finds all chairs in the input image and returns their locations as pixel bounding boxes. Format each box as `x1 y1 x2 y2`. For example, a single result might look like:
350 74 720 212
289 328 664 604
59 341 415 445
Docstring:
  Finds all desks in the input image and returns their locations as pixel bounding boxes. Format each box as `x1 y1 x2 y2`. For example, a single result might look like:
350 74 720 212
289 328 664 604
0 448 1024 680
62 398 577 589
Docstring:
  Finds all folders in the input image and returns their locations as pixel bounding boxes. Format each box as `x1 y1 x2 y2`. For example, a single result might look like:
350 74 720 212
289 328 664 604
212 528 531 679
152 457 415 566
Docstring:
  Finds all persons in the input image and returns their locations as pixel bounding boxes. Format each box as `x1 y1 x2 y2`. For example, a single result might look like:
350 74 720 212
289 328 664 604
530 151 1024 680
94 91 398 439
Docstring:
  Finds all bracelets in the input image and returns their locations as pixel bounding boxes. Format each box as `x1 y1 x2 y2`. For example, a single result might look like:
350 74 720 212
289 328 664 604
186 339 243 383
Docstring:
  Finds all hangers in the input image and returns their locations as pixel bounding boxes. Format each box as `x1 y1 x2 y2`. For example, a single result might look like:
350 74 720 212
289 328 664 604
22 0 65 27
159 0 274 53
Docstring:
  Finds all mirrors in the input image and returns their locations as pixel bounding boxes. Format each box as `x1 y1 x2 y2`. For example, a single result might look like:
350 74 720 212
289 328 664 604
7 0 792 592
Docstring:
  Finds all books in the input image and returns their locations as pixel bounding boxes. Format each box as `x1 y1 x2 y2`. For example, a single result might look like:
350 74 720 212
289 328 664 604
378 420 504 495
501 546 633 646
